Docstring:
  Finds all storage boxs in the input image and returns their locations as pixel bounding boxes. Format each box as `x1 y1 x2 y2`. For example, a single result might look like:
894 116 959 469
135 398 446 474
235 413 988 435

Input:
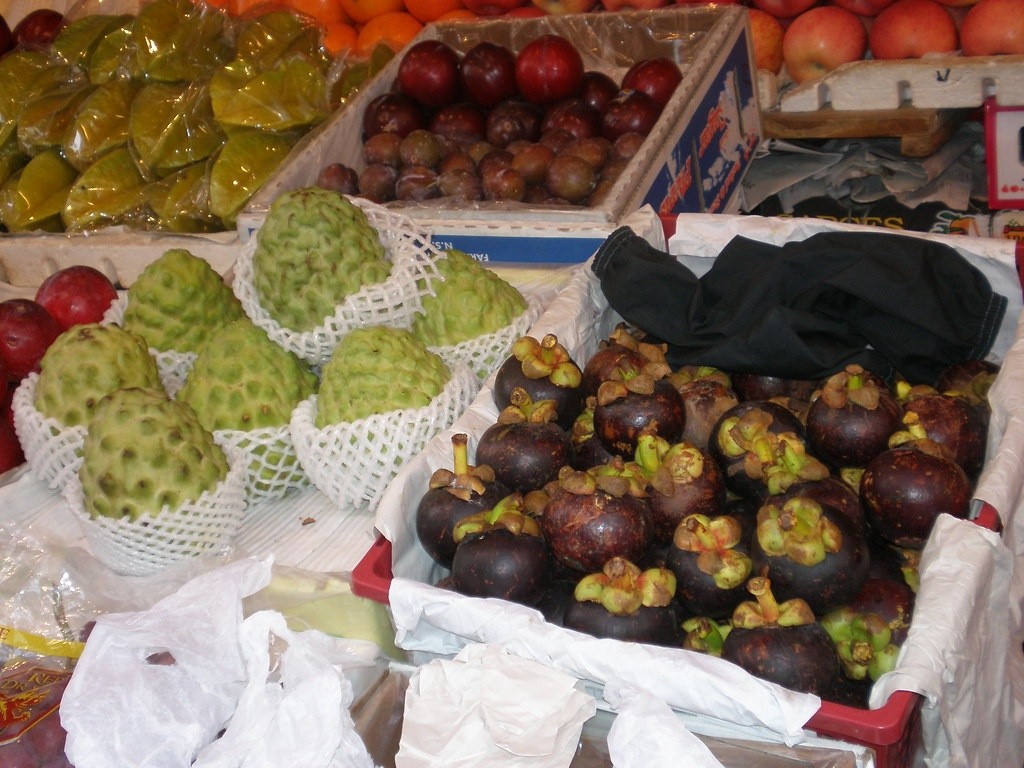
234 6 760 290
1 260 581 675
347 661 877 768
0 33 396 291
351 210 1024 768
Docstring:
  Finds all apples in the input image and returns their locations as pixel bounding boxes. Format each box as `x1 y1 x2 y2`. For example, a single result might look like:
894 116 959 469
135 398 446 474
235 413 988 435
452 0 1024 83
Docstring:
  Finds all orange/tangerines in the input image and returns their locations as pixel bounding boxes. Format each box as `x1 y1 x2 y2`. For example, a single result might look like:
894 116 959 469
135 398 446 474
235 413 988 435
192 0 480 63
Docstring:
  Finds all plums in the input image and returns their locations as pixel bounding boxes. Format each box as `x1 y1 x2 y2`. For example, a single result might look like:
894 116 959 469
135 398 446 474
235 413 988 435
318 35 681 211
0 265 118 478
